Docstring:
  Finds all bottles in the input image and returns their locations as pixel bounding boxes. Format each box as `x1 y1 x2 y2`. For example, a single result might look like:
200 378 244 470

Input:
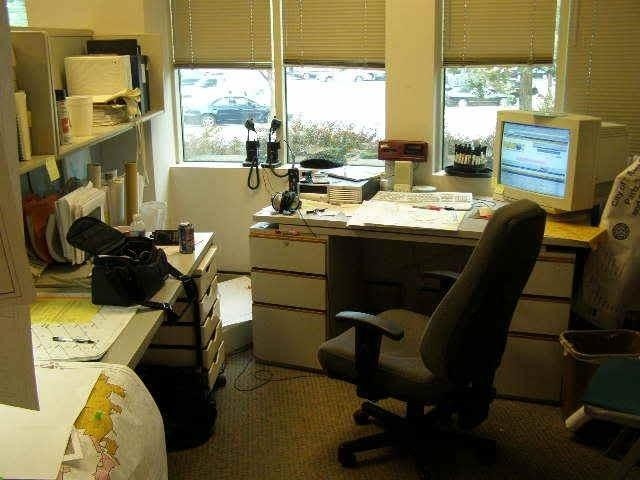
134 214 145 238
55 89 70 144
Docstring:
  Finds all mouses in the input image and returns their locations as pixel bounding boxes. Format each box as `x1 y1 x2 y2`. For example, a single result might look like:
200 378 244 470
478 206 492 217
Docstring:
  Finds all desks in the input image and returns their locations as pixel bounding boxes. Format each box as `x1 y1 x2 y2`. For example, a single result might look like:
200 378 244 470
249 192 604 406
0 230 226 480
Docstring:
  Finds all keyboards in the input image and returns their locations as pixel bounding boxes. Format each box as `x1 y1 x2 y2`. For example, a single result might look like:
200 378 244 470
371 190 473 211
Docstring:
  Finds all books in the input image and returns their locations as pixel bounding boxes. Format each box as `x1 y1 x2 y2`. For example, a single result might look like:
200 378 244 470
55 187 106 265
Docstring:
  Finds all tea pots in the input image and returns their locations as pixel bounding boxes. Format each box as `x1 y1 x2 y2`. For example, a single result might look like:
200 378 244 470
66 95 94 137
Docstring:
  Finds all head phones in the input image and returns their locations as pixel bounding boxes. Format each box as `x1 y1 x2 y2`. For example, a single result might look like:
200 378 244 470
271 191 302 213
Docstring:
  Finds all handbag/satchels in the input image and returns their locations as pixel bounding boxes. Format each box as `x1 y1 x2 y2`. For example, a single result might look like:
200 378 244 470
67 216 198 325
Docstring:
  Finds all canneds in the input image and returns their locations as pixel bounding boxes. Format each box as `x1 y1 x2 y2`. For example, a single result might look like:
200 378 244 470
178 222 195 254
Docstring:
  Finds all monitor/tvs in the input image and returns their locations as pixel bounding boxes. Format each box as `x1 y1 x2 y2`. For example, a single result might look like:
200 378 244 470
492 110 628 215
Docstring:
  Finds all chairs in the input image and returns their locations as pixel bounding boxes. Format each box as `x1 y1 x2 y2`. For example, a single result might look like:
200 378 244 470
316 200 548 472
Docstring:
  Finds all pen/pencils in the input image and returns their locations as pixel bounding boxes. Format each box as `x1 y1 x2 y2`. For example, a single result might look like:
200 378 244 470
53 337 95 343
454 144 487 165
307 210 324 214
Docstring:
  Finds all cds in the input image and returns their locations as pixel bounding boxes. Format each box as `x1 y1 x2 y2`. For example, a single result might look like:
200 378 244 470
532 112 558 118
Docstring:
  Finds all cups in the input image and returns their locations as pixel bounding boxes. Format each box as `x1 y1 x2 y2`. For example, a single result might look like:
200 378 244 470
143 201 168 231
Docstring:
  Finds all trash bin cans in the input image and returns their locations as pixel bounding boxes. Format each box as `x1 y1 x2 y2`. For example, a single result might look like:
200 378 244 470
560 329 640 418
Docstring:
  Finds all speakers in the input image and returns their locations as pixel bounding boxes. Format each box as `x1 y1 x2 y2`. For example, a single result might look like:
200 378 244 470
393 160 413 192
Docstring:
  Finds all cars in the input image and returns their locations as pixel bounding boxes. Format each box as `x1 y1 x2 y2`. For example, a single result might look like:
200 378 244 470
292 66 385 82
445 80 538 106
185 95 271 126
